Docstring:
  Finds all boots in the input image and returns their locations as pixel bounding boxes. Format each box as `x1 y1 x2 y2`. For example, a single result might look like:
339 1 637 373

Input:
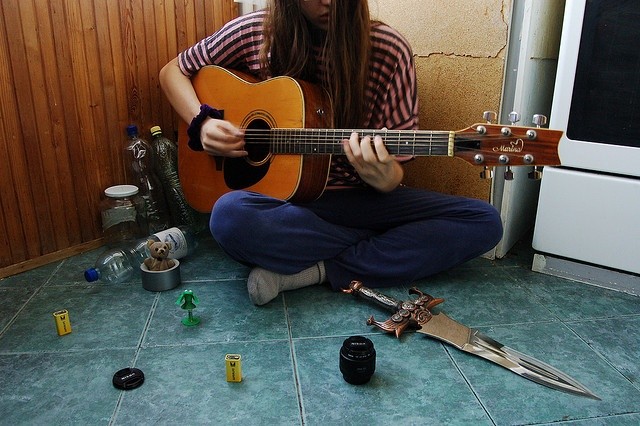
247 259 327 305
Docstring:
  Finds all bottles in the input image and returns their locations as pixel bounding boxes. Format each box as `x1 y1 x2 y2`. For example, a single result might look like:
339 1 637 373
150 125 206 235
121 124 171 237
84 225 197 286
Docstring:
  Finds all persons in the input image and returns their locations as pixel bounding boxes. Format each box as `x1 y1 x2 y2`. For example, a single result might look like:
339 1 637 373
159 0 502 305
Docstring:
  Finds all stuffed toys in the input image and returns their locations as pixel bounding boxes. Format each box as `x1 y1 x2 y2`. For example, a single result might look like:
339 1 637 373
144 239 175 271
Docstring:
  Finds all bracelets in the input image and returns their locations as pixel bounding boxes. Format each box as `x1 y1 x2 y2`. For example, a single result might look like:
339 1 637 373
184 102 226 151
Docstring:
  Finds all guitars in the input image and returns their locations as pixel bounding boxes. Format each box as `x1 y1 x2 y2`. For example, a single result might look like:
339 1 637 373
177 63 566 213
